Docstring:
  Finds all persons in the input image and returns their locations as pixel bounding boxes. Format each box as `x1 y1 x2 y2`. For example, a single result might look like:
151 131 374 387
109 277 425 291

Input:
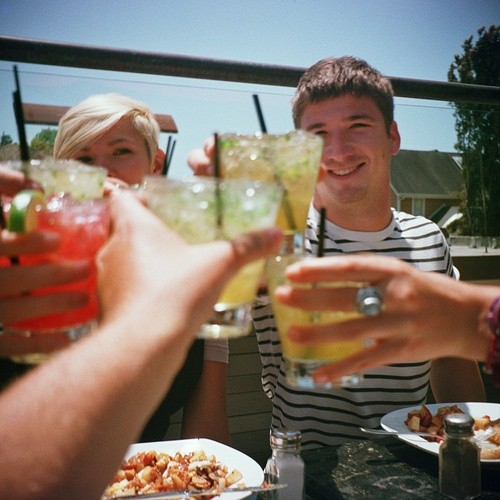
0 191 284 500
274 255 500 384
0 92 229 446
188 56 486 455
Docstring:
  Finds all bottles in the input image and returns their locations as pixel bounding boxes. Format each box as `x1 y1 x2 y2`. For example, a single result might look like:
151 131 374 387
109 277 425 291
260 428 305 500
439 413 481 500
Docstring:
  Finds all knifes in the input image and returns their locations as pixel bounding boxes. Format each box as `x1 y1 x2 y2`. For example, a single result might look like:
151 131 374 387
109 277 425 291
107 484 289 500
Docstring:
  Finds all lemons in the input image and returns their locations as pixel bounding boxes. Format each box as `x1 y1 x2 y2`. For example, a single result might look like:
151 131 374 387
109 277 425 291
8 188 45 233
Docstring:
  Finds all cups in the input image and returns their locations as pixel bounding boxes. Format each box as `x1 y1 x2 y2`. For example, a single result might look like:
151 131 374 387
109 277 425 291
267 252 371 388
0 158 130 364
213 130 325 263
141 175 285 339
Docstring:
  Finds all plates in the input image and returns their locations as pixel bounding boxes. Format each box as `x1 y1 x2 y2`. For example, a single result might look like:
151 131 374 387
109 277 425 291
100 437 264 500
380 402 500 468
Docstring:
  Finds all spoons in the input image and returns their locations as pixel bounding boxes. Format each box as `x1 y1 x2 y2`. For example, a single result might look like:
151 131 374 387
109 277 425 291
358 427 498 446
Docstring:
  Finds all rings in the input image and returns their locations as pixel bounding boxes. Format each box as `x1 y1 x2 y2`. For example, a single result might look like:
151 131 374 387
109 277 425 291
354 285 384 316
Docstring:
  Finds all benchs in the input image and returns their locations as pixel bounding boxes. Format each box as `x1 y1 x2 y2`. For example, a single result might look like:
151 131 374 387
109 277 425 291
164 255 499 469
